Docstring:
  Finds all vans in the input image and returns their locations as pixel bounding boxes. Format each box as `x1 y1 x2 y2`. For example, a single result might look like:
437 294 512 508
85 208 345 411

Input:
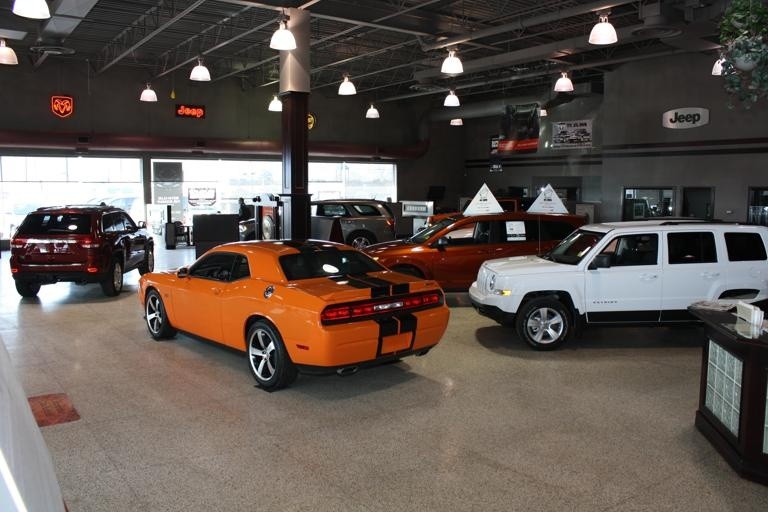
365 210 599 293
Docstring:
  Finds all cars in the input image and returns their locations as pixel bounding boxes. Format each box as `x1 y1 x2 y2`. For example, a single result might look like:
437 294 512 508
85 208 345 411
310 198 395 251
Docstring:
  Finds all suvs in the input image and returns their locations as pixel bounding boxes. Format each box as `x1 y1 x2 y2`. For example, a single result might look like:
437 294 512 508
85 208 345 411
8 203 154 297
470 216 768 352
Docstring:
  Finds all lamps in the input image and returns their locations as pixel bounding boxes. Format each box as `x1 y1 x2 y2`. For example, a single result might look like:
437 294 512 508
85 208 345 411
444 89 460 106
0 0 51 66
139 82 158 102
711 57 736 76
189 58 211 81
268 95 282 112
269 13 297 51
441 47 464 78
337 76 357 96
450 118 464 126
553 72 574 92
365 104 380 119
588 9 619 45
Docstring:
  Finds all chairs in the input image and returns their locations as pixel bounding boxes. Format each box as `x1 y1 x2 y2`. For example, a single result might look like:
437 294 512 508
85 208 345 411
621 242 657 264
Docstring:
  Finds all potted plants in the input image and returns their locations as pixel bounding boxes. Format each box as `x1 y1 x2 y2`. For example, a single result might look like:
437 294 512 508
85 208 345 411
716 0 768 112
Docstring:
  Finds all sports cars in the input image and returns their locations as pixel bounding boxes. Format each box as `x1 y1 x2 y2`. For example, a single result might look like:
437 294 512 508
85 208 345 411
138 238 448 390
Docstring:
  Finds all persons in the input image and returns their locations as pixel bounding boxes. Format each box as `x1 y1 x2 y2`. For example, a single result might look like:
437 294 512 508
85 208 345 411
238 198 251 221
